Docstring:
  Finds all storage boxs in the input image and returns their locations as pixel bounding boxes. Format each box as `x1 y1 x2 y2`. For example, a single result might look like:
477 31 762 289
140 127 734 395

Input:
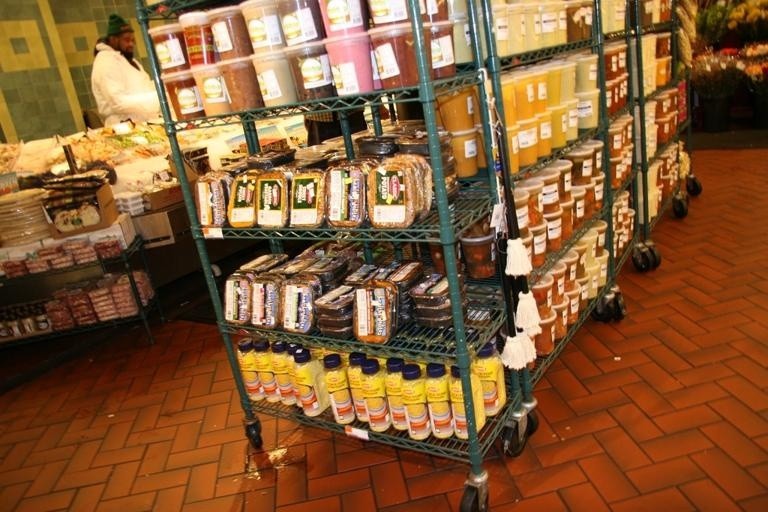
40 183 118 240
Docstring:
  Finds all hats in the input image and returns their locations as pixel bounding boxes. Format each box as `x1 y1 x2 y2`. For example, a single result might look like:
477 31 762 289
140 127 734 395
108 14 134 35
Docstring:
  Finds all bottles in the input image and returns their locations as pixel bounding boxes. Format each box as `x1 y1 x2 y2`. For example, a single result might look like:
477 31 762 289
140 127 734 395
237 324 506 440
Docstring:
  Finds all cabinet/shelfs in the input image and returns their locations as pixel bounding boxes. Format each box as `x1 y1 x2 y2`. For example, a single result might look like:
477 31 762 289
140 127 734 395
0 176 160 346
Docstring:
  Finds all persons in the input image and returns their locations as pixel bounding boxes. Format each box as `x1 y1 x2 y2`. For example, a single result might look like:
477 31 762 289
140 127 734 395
90 13 172 126
